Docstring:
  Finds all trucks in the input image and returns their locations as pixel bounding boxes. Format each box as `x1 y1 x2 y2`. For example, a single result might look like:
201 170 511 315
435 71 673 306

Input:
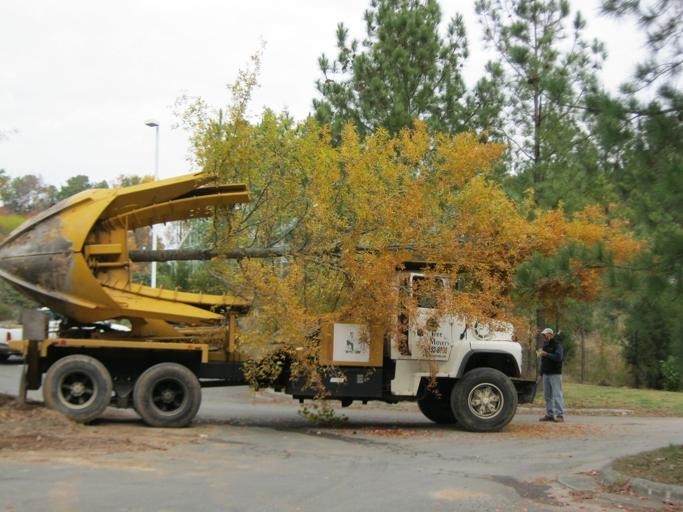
0 258 540 429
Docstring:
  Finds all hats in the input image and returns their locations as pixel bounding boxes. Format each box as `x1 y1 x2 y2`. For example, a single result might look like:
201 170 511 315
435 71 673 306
541 328 553 335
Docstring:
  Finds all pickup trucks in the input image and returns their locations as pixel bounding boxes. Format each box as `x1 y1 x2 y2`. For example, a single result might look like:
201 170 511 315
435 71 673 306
0 306 132 363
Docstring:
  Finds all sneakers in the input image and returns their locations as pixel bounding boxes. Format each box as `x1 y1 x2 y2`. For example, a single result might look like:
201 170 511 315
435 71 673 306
539 416 564 422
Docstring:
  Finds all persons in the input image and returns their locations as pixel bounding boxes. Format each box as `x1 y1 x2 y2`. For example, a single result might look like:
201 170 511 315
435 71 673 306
536 327 566 423
